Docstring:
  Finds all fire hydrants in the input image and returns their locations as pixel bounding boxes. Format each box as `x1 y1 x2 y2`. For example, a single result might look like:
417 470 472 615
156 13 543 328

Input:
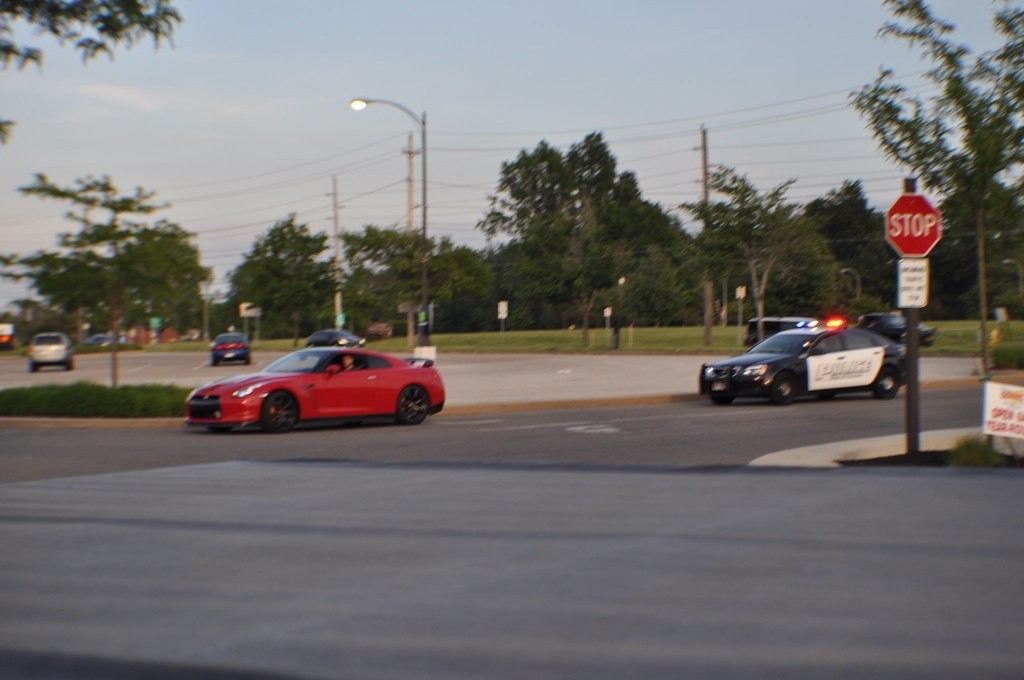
989 326 1002 348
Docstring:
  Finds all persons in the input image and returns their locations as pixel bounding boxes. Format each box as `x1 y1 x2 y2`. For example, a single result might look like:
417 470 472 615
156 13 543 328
341 354 358 371
823 339 838 354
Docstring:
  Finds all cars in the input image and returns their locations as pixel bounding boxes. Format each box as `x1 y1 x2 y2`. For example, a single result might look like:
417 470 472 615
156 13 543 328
209 333 252 367
743 307 844 347
177 343 446 434
82 333 125 348
855 310 939 348
699 319 921 407
304 327 367 349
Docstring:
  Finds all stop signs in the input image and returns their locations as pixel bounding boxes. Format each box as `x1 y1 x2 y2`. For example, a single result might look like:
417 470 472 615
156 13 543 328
883 192 942 259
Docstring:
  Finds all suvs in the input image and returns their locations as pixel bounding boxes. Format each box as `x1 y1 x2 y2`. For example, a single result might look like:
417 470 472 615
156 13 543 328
27 332 75 373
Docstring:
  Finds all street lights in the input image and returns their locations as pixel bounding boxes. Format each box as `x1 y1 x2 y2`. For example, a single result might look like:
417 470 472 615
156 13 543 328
616 276 627 329
351 97 432 347
1001 257 1024 297
840 267 862 299
